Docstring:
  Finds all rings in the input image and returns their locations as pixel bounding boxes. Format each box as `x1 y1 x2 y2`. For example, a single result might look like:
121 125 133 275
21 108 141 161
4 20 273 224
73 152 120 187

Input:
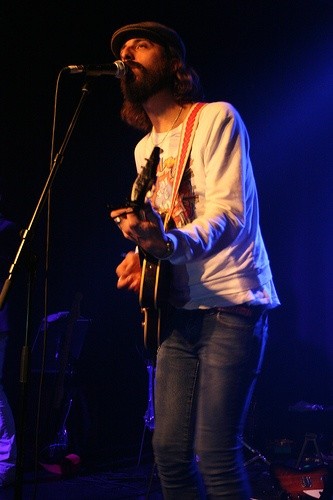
114 215 121 224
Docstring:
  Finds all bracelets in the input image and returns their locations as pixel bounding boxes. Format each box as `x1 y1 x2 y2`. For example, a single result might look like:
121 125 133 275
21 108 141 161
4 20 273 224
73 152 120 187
160 237 173 259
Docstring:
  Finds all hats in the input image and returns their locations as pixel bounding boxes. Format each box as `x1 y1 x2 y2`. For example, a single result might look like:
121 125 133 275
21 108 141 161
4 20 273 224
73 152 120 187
112 21 186 65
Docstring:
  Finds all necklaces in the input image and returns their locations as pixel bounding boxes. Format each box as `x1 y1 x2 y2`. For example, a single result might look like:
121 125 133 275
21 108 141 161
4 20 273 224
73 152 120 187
150 107 182 145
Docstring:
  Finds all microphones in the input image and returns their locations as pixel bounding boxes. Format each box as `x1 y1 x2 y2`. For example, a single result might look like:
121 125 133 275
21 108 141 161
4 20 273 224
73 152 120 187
66 60 129 79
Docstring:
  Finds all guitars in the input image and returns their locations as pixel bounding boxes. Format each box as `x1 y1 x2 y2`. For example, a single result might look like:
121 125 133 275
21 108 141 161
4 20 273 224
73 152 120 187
129 147 167 361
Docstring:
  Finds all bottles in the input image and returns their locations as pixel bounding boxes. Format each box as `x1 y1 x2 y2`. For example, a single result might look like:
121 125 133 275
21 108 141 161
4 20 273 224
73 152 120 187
296 433 323 471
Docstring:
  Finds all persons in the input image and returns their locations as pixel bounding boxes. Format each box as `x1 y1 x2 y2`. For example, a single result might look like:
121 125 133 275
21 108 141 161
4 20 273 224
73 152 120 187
110 21 280 499
